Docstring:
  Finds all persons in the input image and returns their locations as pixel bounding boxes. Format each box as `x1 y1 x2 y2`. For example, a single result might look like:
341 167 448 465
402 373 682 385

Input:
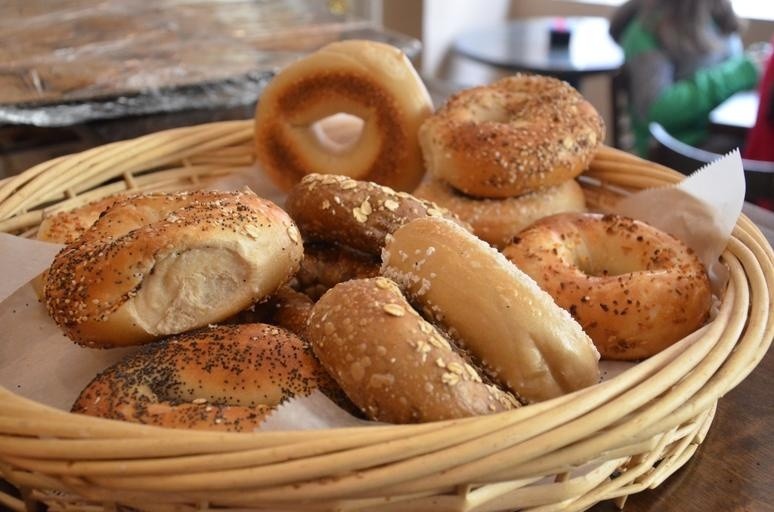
608 0 770 160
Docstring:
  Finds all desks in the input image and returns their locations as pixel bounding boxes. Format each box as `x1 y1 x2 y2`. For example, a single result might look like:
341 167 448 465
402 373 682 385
444 15 630 97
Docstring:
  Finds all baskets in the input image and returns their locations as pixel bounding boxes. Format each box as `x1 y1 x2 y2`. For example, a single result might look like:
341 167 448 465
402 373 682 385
0 119 774 512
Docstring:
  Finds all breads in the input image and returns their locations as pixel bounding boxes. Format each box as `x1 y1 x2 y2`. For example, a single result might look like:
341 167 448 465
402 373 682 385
254 39 435 194
307 274 521 423
71 323 356 435
266 286 359 412
379 215 600 407
412 170 586 252
284 172 474 257
500 211 713 362
296 238 382 301
42 185 305 350
417 75 606 199
37 194 131 242
